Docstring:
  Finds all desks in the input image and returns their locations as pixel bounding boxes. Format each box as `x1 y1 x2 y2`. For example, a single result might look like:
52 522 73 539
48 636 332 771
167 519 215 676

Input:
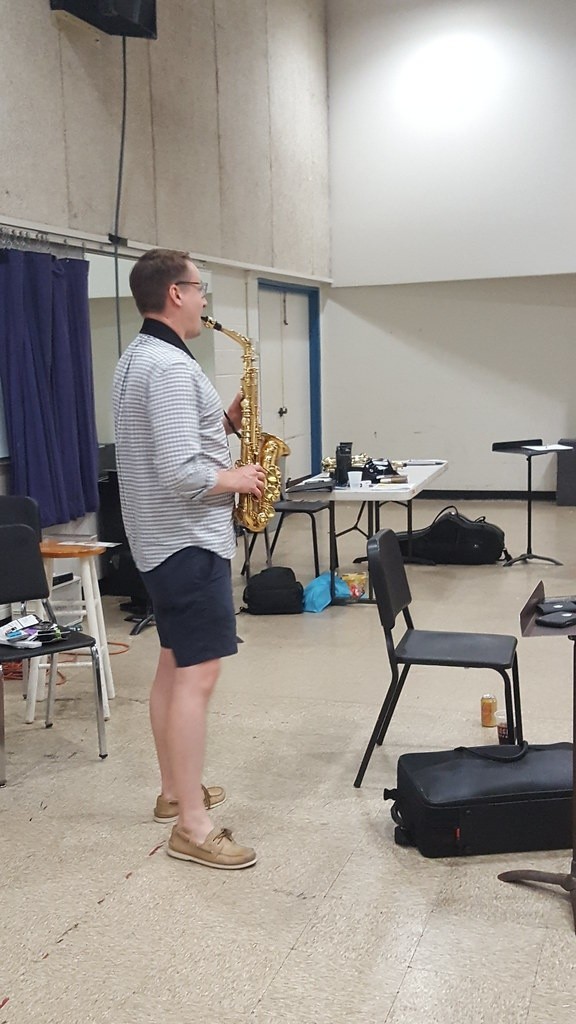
293 459 449 589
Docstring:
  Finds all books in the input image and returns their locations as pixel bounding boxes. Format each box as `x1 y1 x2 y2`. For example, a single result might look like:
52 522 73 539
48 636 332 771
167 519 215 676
380 477 407 483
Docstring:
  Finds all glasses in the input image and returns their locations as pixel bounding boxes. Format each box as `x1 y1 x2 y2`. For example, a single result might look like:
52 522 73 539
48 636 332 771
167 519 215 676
175 280 208 298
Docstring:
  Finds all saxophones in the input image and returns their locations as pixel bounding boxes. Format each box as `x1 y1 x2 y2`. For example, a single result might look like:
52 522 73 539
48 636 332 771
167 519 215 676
198 315 291 532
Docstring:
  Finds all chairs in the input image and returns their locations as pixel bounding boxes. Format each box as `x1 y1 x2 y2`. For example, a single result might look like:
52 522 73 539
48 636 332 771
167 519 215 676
0 522 113 760
239 497 339 585
349 526 528 790
0 494 61 628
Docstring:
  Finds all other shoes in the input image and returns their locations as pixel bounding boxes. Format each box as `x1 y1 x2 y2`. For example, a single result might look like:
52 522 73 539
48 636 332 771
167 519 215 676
153 787 256 869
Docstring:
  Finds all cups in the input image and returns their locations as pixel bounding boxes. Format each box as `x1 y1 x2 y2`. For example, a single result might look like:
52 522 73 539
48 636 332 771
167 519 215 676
493 710 522 746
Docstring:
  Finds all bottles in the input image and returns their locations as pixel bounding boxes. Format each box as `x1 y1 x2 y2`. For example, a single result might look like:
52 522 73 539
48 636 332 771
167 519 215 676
480 694 497 728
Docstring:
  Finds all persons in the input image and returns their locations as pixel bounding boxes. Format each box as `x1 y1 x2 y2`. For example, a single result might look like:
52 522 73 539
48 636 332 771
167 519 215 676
111 245 268 872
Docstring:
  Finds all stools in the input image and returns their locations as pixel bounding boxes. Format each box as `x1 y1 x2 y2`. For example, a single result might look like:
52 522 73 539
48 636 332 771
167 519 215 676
23 539 118 725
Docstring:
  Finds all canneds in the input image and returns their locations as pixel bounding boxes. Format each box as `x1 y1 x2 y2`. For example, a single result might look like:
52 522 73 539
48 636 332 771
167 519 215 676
481 694 498 727
